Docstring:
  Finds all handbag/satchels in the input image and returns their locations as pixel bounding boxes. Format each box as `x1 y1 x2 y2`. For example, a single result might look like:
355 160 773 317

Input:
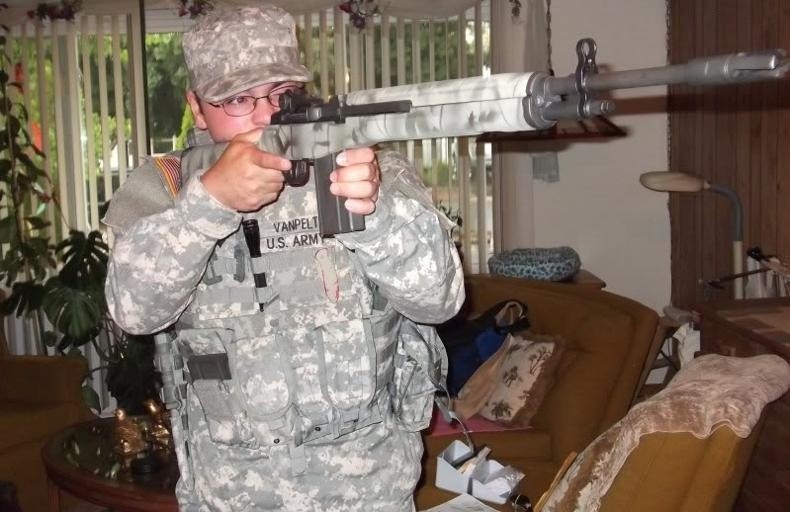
447 298 530 423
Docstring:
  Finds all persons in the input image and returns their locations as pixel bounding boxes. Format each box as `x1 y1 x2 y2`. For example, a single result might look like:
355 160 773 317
100 3 466 512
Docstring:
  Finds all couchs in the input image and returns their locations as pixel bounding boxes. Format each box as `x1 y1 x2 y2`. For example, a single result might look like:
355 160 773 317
0 355 83 512
414 274 665 483
414 354 788 509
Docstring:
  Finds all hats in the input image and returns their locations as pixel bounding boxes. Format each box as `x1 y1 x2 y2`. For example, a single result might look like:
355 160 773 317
179 5 315 103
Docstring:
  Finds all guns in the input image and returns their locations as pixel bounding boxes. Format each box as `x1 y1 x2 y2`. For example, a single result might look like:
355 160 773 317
180 38 788 236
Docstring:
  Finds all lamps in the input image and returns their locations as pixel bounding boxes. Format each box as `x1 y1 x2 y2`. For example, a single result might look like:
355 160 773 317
639 172 744 305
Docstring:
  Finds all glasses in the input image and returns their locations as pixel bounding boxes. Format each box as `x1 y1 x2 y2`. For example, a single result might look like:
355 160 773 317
203 84 302 118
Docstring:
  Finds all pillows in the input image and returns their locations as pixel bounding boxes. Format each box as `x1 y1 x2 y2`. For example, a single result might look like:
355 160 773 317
478 330 569 428
